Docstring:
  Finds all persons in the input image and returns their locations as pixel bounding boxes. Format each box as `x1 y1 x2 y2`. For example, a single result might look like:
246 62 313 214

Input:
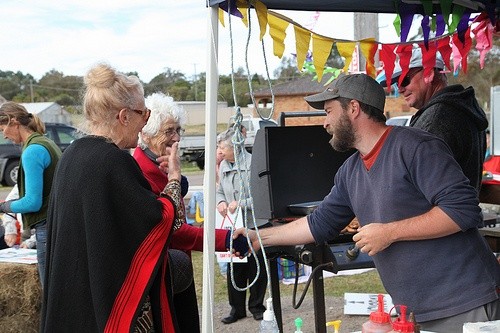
130 72 500 333
41 60 182 333
216 127 273 325
0 101 62 294
374 47 489 205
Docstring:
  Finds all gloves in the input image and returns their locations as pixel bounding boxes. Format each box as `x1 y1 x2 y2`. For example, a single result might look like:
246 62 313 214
225 230 253 257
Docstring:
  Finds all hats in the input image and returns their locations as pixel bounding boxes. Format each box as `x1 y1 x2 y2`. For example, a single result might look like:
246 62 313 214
374 47 454 86
304 72 386 113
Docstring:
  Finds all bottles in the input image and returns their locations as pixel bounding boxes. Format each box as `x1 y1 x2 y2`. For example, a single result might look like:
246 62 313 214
362 295 394 333
389 306 415 333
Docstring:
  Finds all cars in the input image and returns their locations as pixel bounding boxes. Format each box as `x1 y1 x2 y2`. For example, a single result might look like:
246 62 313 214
0 122 91 187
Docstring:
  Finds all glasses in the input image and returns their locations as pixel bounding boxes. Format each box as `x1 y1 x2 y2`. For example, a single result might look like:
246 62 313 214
158 127 185 138
116 106 151 123
396 68 424 88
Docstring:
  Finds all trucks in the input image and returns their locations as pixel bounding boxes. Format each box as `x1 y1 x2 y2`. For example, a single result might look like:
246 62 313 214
176 114 279 172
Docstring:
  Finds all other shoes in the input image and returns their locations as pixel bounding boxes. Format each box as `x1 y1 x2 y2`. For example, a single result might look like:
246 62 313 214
221 314 245 323
253 311 263 320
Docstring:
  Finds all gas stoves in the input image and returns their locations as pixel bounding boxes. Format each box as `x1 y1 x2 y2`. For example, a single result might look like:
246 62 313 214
274 215 375 274
